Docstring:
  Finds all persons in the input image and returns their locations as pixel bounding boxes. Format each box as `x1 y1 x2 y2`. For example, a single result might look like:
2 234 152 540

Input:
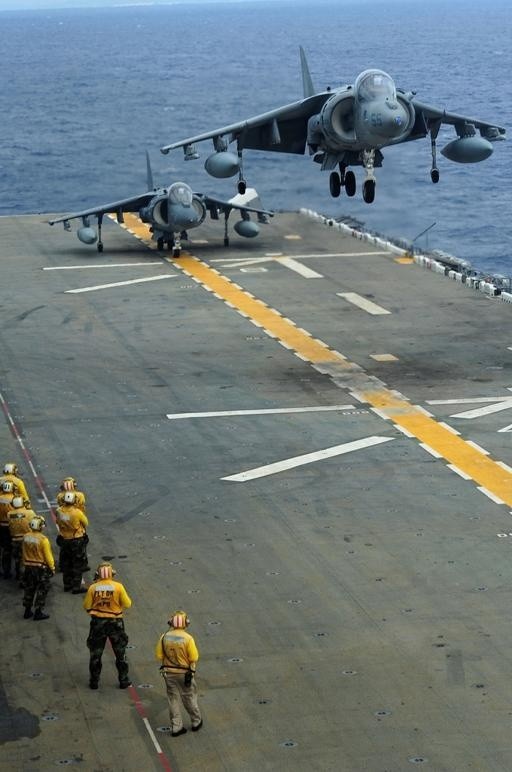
0 462 33 509
18 514 57 622
81 560 134 691
5 495 39 588
154 609 204 738
0 481 32 583
56 476 91 573
55 491 89 594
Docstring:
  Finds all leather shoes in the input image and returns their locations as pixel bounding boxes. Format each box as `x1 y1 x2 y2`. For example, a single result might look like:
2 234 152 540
191 720 203 731
172 727 187 737
72 588 87 594
120 680 132 690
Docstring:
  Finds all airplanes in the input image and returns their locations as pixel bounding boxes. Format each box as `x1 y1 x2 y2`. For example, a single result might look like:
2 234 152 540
39 146 281 262
158 42 508 206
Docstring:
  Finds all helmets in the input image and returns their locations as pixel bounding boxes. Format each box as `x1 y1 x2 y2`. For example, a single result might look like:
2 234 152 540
97 562 114 579
170 609 189 629
2 463 18 476
63 491 77 505
62 476 76 491
2 481 15 494
30 516 45 532
11 496 26 509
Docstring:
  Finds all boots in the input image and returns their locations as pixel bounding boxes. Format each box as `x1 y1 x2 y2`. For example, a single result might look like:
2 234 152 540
23 609 35 619
33 607 51 621
